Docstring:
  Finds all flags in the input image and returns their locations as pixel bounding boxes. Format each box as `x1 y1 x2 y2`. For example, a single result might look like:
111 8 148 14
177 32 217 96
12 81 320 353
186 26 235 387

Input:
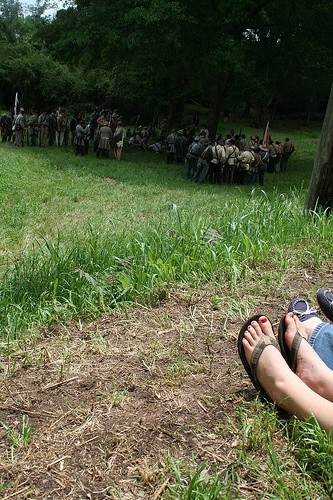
263 121 269 145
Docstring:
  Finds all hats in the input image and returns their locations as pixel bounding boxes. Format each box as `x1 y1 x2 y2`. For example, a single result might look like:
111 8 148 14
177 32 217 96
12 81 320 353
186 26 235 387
259 144 268 151
275 141 280 144
228 139 234 144
177 130 183 135
218 139 225 145
195 137 200 140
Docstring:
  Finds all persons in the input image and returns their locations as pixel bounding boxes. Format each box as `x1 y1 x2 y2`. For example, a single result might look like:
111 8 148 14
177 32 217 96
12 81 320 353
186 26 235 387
69 105 126 162
288 288 333 371
185 129 295 187
126 112 209 164
0 107 69 148
237 312 333 435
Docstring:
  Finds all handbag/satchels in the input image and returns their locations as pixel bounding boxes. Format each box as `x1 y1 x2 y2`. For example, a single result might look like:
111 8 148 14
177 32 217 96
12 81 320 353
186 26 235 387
248 167 256 175
239 162 246 168
274 158 279 163
115 132 122 142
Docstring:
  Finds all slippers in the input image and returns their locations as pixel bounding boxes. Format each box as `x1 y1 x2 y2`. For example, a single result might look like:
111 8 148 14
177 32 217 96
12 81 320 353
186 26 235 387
278 315 311 373
237 314 297 419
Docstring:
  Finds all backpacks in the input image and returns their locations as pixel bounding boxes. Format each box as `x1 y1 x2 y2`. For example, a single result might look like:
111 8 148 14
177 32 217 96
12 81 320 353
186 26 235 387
250 151 261 168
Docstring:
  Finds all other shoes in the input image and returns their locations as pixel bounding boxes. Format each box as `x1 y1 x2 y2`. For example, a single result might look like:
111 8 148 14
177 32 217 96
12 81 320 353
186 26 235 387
316 288 333 324
288 298 318 322
